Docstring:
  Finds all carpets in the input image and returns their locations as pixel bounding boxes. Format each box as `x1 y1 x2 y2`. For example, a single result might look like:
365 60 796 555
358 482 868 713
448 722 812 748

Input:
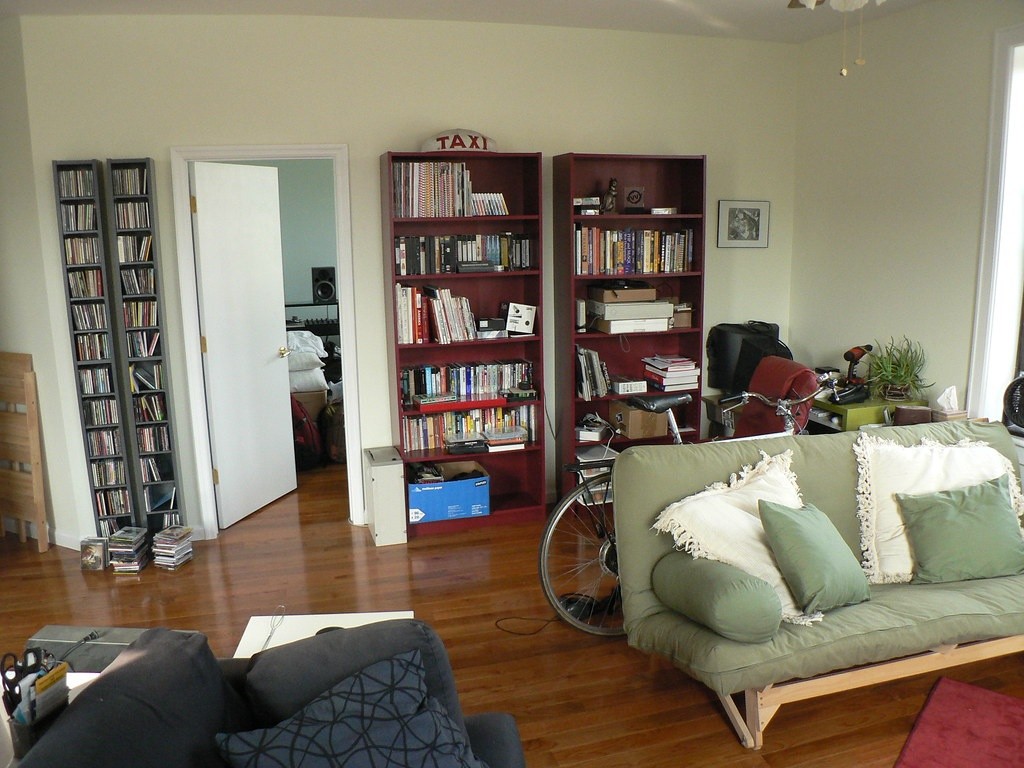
893 676 1024 768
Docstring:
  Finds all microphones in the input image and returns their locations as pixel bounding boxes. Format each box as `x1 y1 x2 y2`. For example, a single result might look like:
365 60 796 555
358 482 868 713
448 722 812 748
844 344 873 361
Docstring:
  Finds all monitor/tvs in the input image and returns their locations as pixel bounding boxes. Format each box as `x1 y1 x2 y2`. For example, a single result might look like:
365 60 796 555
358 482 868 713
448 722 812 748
707 324 791 394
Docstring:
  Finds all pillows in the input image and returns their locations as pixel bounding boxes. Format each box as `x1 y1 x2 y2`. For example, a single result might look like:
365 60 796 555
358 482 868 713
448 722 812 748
757 499 872 617
894 472 1024 584
649 448 826 628
213 649 483 768
852 431 1024 584
651 550 782 643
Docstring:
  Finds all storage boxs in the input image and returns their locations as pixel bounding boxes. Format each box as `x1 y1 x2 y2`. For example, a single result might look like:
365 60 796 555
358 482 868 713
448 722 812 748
673 312 692 328
575 444 621 507
588 317 671 335
587 285 657 302
611 375 647 395
587 300 674 321
477 331 509 338
498 302 536 334
408 461 491 525
478 318 505 331
651 208 677 215
609 399 668 441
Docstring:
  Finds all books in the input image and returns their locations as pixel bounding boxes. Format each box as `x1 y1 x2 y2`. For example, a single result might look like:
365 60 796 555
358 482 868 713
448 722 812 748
398 357 537 406
575 343 611 401
643 354 700 392
392 162 509 218
395 282 477 344
573 221 693 275
394 231 529 276
113 168 180 529
402 404 535 454
80 526 194 574
58 168 131 537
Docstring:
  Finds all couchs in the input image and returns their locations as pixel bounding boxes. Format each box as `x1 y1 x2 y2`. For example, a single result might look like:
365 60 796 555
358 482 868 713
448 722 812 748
611 417 1024 768
13 620 526 768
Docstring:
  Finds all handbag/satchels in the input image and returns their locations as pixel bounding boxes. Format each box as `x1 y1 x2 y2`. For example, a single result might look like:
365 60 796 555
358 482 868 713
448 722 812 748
290 394 323 469
319 399 346 463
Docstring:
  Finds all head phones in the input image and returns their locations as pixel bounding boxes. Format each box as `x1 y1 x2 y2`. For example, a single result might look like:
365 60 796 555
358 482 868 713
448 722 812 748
556 593 598 621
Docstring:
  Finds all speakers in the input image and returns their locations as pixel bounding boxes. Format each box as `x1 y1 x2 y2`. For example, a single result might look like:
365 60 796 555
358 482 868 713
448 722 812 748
311 266 336 303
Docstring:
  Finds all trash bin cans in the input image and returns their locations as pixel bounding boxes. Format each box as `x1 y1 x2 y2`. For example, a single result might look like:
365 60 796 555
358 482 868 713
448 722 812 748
363 446 407 547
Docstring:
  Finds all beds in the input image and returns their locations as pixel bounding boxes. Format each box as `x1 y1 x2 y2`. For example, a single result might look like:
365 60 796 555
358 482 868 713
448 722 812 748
287 330 332 420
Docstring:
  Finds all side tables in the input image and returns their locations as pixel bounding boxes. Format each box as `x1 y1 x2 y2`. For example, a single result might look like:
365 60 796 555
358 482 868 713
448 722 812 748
231 610 415 660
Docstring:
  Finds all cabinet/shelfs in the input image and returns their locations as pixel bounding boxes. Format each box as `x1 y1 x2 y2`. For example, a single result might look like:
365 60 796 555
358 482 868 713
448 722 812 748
553 151 708 517
285 300 340 346
52 157 189 561
380 152 546 536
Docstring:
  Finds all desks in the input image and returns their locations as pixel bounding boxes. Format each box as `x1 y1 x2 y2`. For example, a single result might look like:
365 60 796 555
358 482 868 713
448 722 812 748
810 385 929 432
702 394 743 428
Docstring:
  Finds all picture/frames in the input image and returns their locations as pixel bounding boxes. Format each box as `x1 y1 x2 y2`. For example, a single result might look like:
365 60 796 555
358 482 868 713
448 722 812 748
717 199 771 248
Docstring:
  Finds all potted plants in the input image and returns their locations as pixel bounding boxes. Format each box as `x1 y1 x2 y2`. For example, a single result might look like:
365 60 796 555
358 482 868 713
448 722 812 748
858 333 936 402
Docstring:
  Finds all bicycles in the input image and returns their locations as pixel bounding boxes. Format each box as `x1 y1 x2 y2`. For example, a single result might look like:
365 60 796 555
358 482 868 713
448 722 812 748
538 371 848 639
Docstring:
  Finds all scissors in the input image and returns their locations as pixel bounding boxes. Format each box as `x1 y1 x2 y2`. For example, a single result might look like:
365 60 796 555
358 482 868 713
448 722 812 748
0 648 40 703
